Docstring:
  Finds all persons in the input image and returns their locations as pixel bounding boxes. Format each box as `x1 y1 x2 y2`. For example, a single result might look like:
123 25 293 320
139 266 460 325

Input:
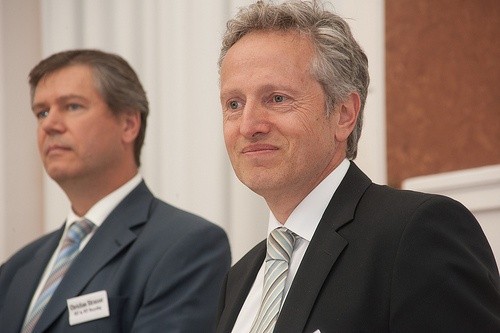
0 50 231 333
215 0 500 333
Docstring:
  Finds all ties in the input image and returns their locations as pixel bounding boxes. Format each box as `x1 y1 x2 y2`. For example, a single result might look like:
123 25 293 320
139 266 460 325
21 220 95 333
249 227 301 333
